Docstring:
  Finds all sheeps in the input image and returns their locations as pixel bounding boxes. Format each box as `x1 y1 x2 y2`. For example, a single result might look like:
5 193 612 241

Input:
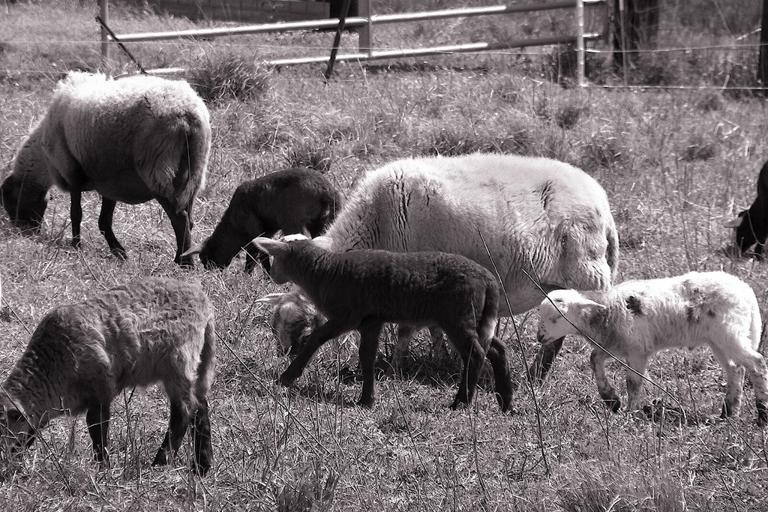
179 167 343 282
0 70 213 271
536 271 768 429
253 151 620 387
723 160 768 262
251 233 516 417
0 276 218 484
